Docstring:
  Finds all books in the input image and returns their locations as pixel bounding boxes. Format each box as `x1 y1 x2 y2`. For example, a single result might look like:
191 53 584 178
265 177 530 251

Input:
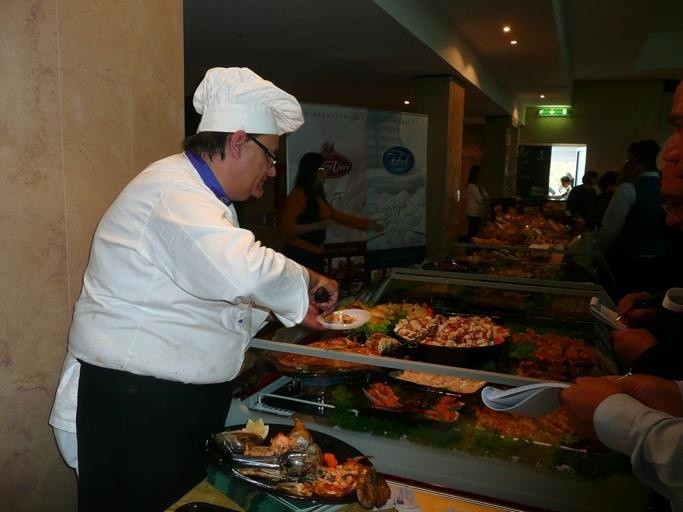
586 296 626 329
478 382 572 418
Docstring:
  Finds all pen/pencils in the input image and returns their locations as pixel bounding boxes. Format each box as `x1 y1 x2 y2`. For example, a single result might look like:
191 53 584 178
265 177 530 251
615 371 633 382
616 316 621 321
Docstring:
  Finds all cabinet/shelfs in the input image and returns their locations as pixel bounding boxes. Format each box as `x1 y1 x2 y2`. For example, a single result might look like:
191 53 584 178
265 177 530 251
283 239 369 302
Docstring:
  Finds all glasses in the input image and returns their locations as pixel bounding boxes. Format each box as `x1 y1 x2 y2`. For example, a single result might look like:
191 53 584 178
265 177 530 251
246 133 277 166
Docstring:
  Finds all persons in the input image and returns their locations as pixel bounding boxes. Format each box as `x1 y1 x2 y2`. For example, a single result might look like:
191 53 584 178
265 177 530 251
276 151 383 275
565 170 599 216
597 137 669 303
544 174 573 201
558 72 683 511
45 64 343 512
614 285 682 385
463 162 491 242
590 171 620 232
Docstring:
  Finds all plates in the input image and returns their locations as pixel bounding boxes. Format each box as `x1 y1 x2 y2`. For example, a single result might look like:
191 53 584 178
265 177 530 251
203 422 375 505
316 308 371 331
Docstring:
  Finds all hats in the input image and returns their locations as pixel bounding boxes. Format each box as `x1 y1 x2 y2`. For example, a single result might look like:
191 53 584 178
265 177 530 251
190 63 305 136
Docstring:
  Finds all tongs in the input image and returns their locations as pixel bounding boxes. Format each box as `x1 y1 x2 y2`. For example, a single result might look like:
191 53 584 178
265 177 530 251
213 451 319 481
